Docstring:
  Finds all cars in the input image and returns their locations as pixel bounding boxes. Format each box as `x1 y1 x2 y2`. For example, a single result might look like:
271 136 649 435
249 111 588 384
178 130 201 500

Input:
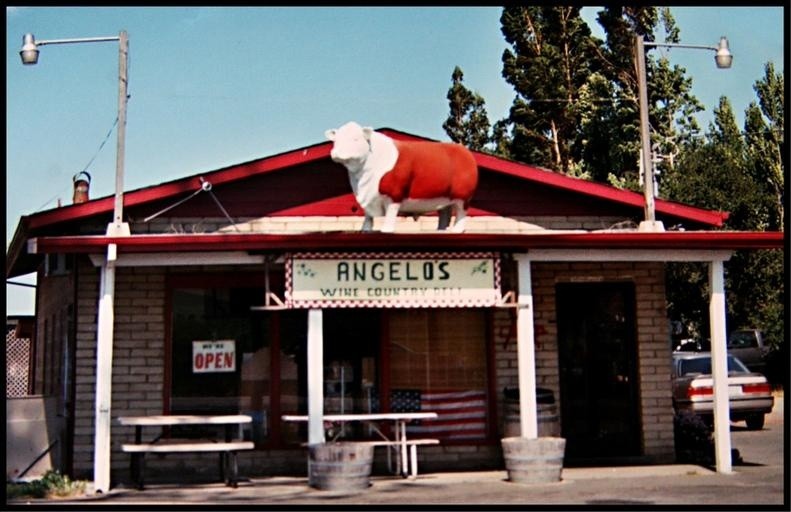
672 327 779 429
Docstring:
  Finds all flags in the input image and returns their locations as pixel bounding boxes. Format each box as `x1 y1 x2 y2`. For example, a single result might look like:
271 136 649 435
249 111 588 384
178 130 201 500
366 386 489 441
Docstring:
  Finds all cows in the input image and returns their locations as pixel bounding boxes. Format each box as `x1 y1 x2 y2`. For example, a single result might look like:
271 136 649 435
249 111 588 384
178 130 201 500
324 120 478 234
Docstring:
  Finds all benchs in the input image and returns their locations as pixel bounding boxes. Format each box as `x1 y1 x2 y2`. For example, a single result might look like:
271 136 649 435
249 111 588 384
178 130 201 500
119 441 257 489
303 437 442 476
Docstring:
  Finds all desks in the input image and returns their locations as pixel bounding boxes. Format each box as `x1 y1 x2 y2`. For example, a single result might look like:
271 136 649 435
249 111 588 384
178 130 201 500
115 413 257 489
281 411 438 473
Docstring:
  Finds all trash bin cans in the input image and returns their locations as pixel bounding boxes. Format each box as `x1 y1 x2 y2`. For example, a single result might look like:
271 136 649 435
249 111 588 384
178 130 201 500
677 400 693 416
503 390 559 435
